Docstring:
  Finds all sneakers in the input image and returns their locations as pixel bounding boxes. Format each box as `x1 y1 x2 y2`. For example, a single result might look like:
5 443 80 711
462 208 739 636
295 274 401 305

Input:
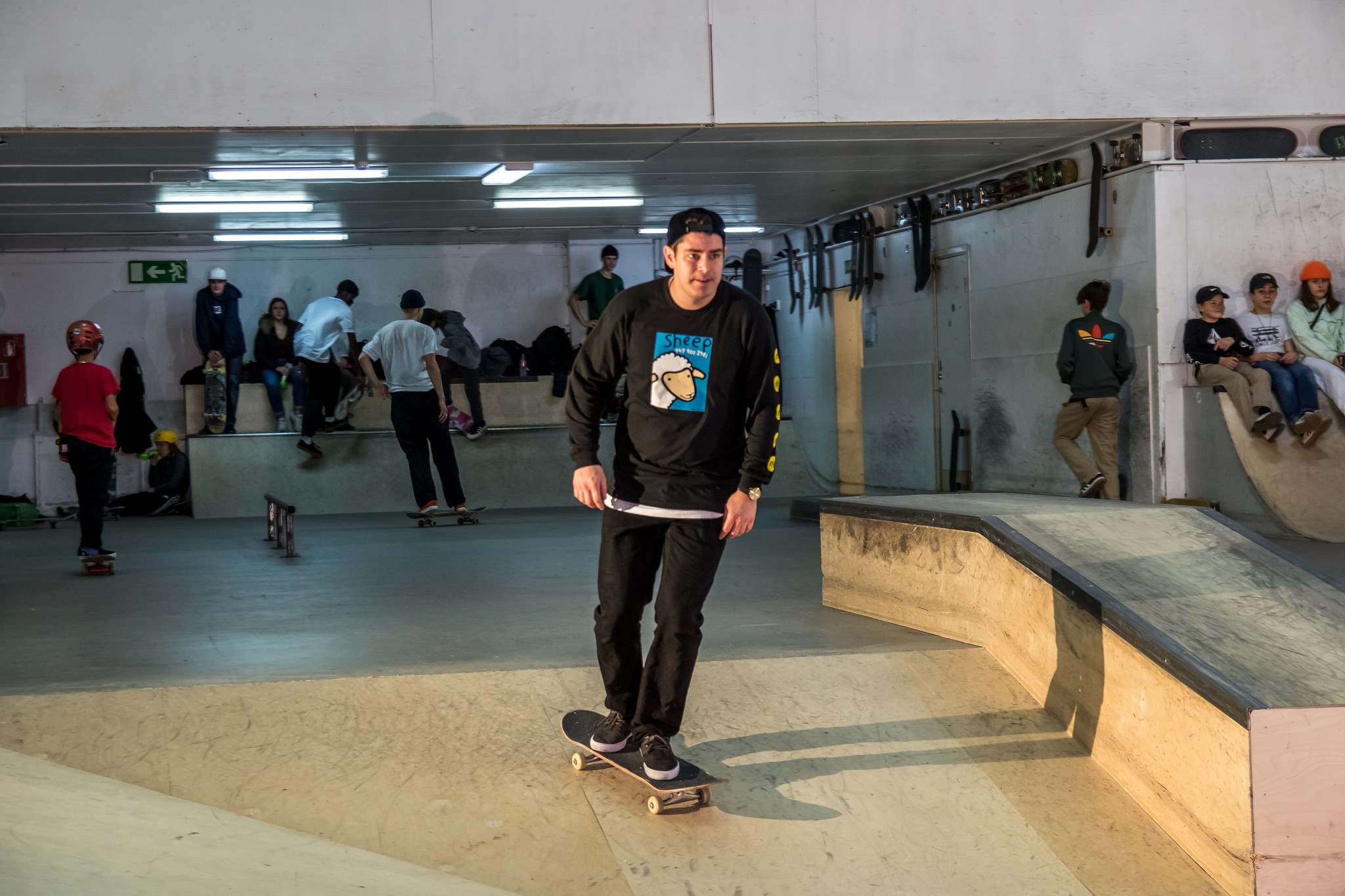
466 420 487 440
590 710 635 752
1293 412 1322 435
297 438 324 455
1302 418 1333 447
1077 473 1106 497
290 411 302 432
455 504 468 511
1255 411 1283 430
446 400 454 407
277 415 288 430
420 500 439 513
640 734 682 780
78 545 117 561
1263 423 1286 440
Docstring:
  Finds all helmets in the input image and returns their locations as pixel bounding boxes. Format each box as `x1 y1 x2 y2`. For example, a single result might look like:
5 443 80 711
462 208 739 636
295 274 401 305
155 428 180 445
67 321 104 352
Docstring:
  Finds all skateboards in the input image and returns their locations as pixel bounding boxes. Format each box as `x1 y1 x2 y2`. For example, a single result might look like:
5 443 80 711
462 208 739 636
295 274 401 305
1084 141 1115 258
740 248 764 302
1109 132 1142 168
1175 127 1299 162
200 350 228 434
407 506 488 527
802 193 934 310
1318 124 1345 159
447 405 487 438
780 232 802 315
333 374 377 420
937 157 1078 218
0 511 77 529
561 704 727 813
947 409 968 492
166 500 188 515
72 507 127 520
78 549 118 576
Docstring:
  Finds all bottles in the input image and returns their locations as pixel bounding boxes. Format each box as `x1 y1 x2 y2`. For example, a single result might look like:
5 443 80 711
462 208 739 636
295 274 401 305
520 354 527 376
141 451 159 460
281 375 287 387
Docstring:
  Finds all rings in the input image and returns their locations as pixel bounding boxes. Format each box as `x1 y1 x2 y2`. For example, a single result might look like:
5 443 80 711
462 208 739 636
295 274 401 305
445 414 448 416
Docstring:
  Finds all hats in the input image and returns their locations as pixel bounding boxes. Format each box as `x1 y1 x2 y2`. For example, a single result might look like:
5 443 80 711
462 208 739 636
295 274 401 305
1195 286 1231 303
399 290 426 309
663 209 727 276
207 266 229 281
601 244 621 258
1300 262 1333 281
1249 274 1280 290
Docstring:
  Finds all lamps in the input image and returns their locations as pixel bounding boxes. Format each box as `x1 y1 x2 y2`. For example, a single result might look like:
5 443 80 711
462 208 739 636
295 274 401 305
492 196 645 209
207 166 389 181
154 201 314 214
213 233 349 242
637 226 766 235
481 164 534 186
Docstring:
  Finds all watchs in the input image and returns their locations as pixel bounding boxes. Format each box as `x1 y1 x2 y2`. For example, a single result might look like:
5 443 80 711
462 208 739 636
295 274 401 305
737 486 762 501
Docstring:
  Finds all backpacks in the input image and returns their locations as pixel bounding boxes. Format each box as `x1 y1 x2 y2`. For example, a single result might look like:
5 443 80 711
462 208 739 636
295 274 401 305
176 326 572 394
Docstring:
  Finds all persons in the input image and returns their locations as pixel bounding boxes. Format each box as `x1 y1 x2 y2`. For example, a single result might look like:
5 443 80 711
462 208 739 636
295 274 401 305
1285 261 1345 417
357 289 472 513
566 207 783 783
1184 285 1285 442
293 279 360 457
104 429 190 517
570 245 625 424
194 267 246 434
254 296 304 433
418 308 488 440
1052 278 1131 501
1232 272 1332 448
320 345 366 434
52 320 121 559
